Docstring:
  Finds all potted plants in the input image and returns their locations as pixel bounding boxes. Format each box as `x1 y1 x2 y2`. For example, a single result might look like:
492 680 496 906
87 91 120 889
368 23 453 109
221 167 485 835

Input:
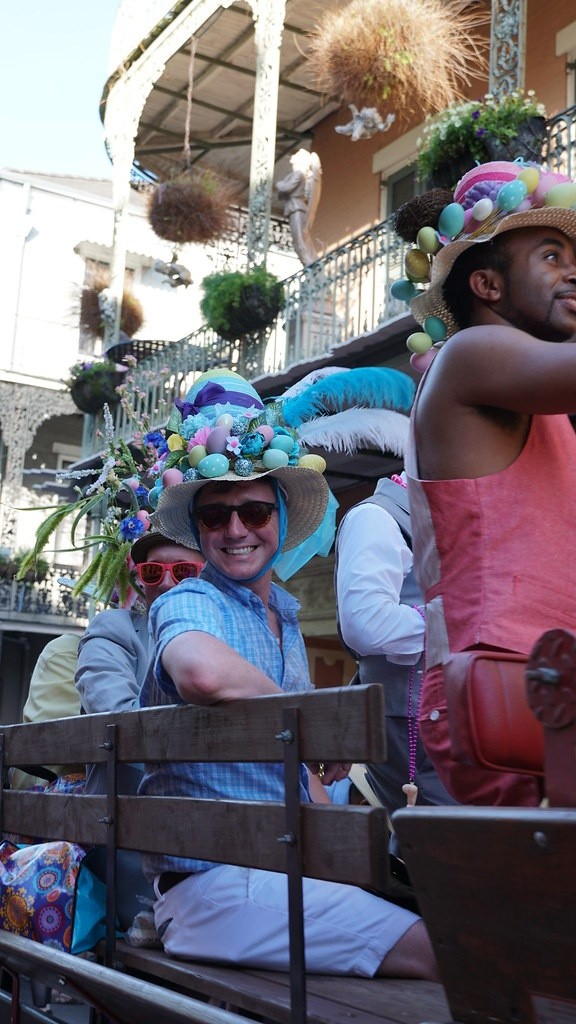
200 261 285 342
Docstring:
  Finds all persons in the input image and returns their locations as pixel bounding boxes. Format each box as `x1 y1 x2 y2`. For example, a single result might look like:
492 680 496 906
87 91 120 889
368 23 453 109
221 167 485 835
392 158 576 808
136 459 438 980
331 469 457 807
10 530 206 797
276 147 318 266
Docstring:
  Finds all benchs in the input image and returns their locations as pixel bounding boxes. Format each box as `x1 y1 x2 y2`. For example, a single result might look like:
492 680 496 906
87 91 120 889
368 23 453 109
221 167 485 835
0 627 576 1024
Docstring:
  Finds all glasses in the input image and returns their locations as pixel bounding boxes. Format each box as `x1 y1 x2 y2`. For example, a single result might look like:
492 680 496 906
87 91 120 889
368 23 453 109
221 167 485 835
135 561 208 586
192 498 280 531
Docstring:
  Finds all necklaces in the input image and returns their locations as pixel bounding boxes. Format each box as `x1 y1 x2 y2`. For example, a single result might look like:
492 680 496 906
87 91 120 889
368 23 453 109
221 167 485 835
391 474 407 488
401 602 427 806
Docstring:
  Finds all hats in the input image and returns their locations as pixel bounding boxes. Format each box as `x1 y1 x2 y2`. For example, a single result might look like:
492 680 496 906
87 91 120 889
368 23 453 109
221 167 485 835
146 367 417 581
160 366 265 434
17 400 184 607
390 161 576 372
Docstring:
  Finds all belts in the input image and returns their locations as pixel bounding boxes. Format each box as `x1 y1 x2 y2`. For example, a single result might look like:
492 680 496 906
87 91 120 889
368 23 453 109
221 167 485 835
158 872 190 895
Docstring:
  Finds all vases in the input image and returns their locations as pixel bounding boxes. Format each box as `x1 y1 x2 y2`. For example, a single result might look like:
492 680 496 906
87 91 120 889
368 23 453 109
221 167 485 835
434 117 545 189
71 372 126 413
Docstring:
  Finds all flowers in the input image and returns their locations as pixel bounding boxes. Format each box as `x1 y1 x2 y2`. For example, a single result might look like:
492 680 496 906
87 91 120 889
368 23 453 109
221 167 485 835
0 367 299 611
60 359 116 390
408 87 545 183
